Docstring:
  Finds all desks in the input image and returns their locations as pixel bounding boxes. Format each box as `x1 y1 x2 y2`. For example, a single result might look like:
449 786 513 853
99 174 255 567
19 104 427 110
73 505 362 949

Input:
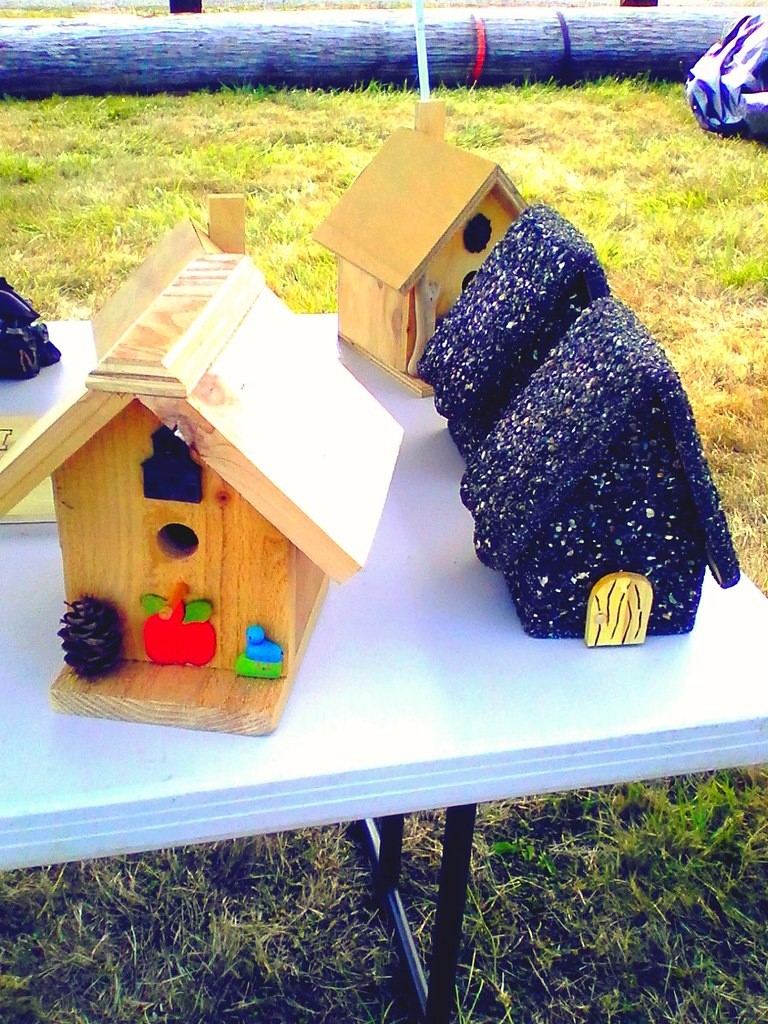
0 311 768 1024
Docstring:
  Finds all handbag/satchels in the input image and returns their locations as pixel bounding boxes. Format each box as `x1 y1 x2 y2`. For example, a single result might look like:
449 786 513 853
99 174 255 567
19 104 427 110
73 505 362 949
687 14 768 139
